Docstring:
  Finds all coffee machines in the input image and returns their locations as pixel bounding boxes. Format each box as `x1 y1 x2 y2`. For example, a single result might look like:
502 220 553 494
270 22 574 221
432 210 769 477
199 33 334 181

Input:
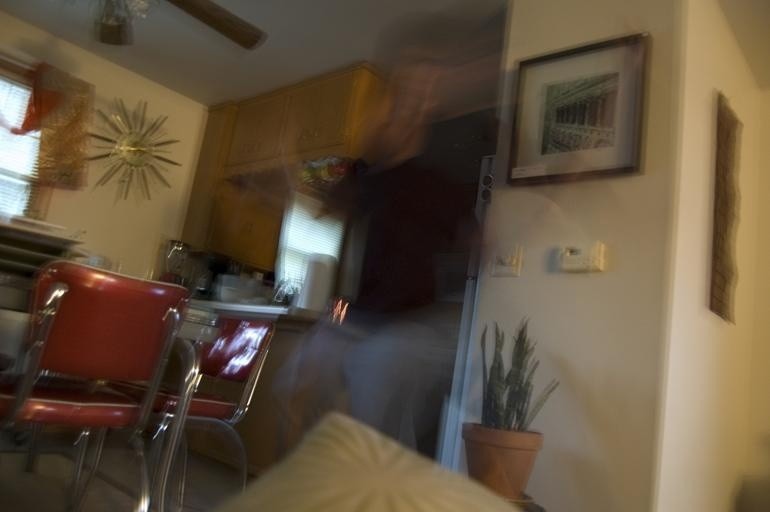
189 249 243 301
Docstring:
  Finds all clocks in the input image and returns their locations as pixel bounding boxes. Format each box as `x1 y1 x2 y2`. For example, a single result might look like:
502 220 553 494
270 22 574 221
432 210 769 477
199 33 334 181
83 97 183 201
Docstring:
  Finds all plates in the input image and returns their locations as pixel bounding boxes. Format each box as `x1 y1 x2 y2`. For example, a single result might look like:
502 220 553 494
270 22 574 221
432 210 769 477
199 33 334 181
1 213 93 279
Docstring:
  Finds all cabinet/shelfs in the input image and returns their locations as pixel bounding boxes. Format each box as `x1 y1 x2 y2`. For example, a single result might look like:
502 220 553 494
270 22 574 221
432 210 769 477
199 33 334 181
224 61 391 189
180 181 287 271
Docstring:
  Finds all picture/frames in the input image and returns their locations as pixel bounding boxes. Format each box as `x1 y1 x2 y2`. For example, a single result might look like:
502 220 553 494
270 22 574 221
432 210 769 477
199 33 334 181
505 29 653 185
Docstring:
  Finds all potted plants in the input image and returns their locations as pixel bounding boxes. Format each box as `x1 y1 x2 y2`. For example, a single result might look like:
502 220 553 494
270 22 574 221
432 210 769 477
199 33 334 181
462 316 560 502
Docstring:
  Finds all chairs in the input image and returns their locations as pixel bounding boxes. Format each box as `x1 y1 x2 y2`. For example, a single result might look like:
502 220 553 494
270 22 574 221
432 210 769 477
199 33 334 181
77 281 301 512
